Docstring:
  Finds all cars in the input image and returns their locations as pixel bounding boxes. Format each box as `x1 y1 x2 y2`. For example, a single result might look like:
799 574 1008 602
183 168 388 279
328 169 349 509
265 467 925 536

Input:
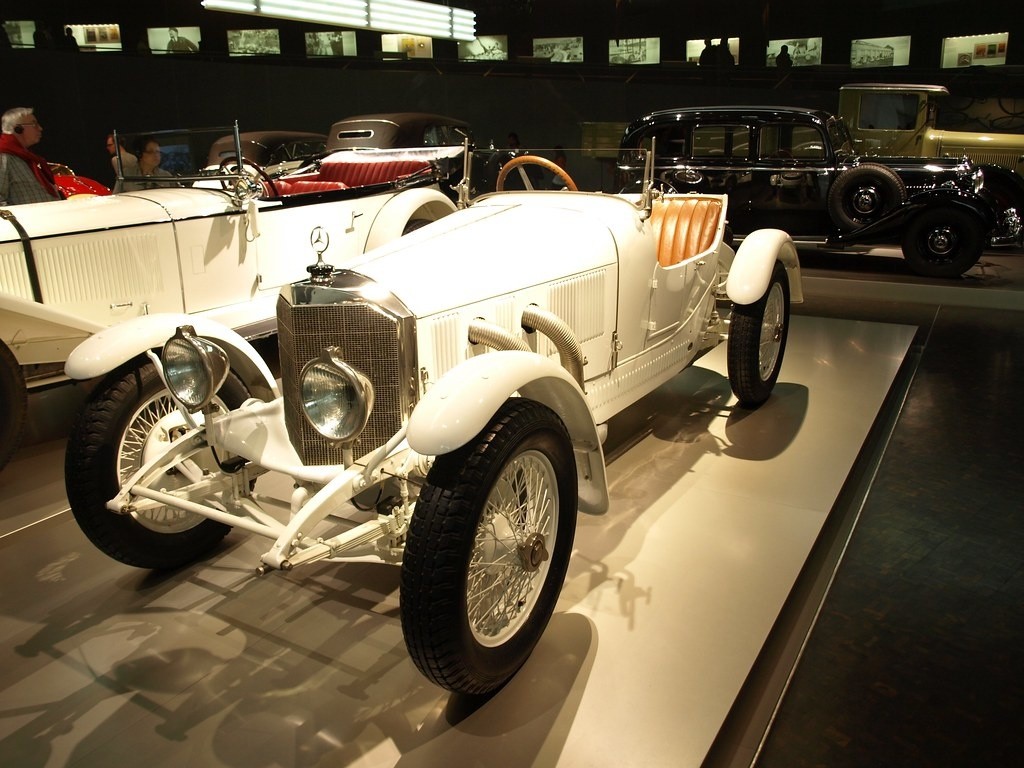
611 104 1024 282
62 122 804 698
706 83 1024 181
0 108 475 471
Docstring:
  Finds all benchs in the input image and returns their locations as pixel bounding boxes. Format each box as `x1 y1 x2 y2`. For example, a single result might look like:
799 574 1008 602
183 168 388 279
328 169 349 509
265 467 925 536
319 145 465 186
617 193 728 266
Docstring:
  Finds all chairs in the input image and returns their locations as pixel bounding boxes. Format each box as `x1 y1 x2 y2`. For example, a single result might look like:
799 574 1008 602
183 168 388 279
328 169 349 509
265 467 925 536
261 180 349 196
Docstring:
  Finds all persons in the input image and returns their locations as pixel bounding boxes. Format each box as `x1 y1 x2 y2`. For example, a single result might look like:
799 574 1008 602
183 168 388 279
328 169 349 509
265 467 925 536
554 146 567 168
166 28 198 53
63 28 78 48
33 21 56 49
776 45 792 71
106 133 136 175
699 38 735 65
0 108 62 205
113 138 182 194
136 32 152 55
0 15 12 48
122 41 129 55
507 133 527 149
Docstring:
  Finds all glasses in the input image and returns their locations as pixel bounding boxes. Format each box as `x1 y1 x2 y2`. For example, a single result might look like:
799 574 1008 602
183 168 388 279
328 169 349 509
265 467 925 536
145 149 160 154
18 122 38 128
107 142 116 147
